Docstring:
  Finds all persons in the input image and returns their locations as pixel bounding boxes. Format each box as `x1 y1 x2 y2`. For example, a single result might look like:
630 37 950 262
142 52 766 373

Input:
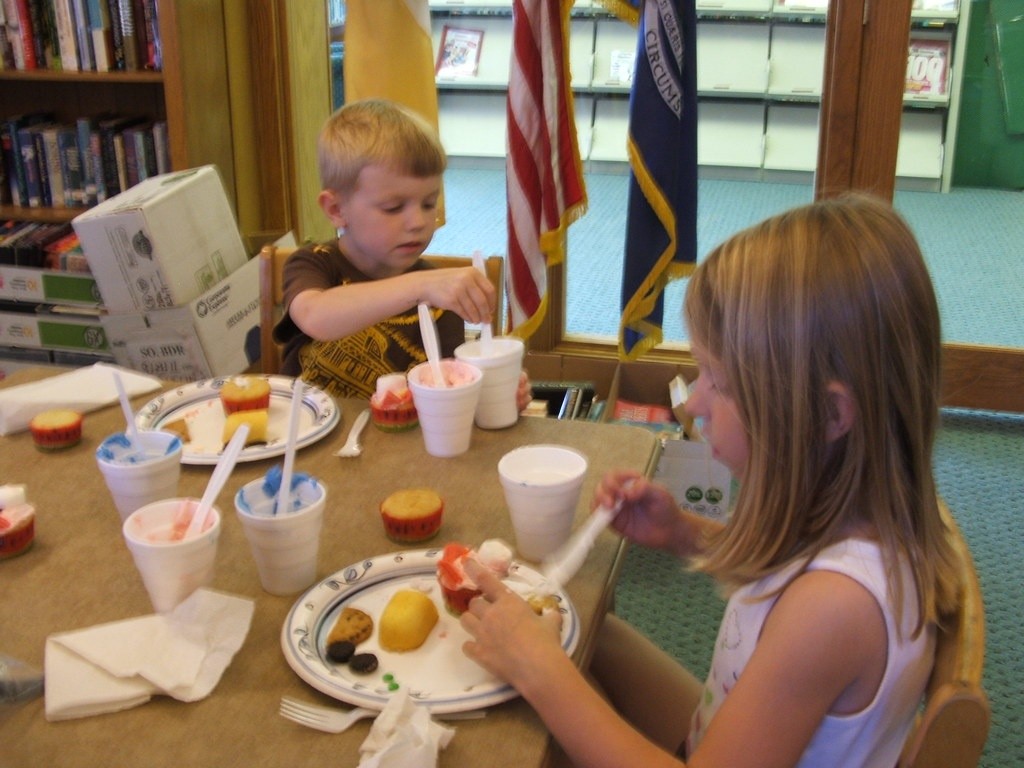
278 100 536 415
453 185 965 768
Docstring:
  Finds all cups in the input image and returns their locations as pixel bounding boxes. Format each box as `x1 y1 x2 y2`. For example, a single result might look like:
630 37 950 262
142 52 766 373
122 499 220 612
407 359 482 457
96 429 182 525
455 338 524 429
496 444 589 564
234 473 328 597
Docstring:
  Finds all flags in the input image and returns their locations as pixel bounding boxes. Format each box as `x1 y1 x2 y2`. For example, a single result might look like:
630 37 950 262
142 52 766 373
598 0 699 362
500 1 594 344
339 0 453 233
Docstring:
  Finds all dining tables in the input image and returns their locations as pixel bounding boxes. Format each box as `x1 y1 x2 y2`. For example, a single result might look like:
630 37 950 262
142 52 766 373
2 357 663 767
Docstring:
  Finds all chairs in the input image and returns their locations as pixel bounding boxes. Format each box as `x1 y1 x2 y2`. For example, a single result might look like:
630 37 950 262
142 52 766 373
254 251 506 396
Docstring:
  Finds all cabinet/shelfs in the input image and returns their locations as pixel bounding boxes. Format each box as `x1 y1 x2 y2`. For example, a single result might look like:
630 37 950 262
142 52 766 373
0 0 234 355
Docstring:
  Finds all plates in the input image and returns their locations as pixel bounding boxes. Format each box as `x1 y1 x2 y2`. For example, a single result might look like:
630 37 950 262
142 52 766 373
279 545 579 712
128 373 339 464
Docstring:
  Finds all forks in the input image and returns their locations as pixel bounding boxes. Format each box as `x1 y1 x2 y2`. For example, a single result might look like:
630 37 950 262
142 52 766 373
281 697 486 733
333 407 372 459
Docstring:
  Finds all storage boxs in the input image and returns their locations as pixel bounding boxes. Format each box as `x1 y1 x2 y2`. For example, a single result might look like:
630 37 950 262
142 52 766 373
69 164 248 315
100 228 309 388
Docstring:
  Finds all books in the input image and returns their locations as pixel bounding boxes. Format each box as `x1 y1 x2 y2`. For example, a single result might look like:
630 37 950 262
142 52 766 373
1 0 159 73
524 377 739 531
904 38 948 99
433 22 485 85
1 107 176 206
0 221 114 362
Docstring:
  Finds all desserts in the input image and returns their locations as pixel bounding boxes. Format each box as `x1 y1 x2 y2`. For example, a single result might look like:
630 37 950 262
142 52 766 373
368 371 421 432
31 409 84 451
0 483 36 560
214 376 272 414
379 489 448 543
